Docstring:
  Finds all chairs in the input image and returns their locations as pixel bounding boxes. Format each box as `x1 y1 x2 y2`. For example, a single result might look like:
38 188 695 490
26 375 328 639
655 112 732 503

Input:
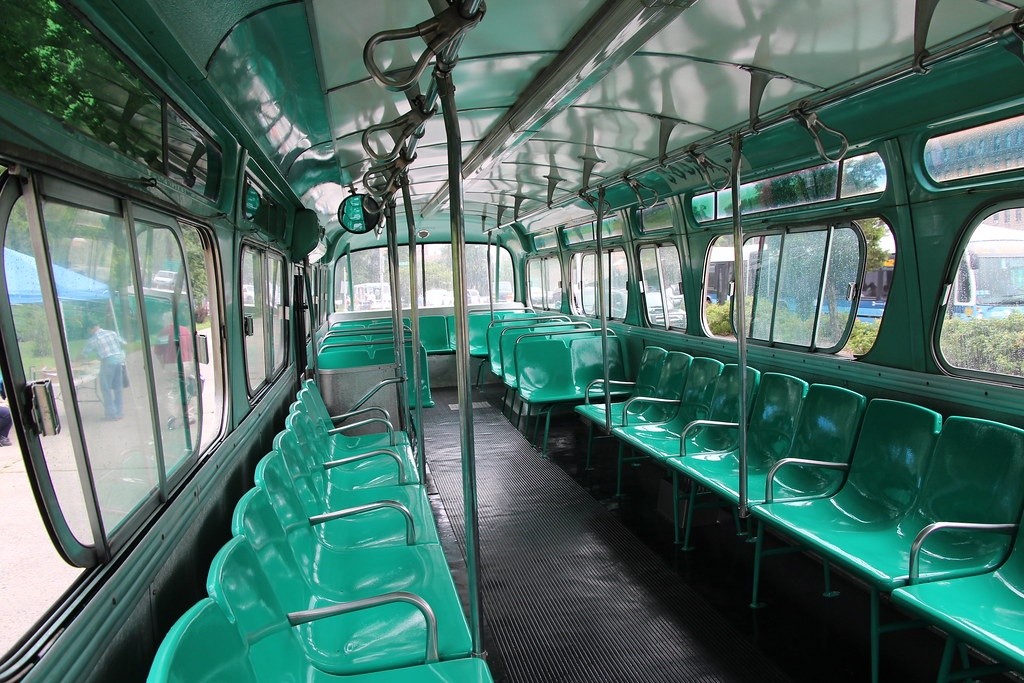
146 308 1024 683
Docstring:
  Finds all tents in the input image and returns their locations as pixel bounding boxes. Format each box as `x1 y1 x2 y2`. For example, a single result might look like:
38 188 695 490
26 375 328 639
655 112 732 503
4 245 134 396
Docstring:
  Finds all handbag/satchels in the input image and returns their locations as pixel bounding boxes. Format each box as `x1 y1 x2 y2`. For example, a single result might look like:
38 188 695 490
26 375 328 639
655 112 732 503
111 365 128 389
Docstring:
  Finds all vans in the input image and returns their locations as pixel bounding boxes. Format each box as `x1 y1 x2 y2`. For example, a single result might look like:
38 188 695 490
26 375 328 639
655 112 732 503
154 271 178 289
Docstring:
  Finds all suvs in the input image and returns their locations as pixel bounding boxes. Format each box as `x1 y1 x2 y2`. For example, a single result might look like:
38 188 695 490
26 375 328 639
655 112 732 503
590 287 686 329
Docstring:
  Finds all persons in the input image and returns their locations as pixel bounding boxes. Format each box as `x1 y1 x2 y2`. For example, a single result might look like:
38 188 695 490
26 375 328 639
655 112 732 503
154 312 197 429
0 376 13 446
72 319 127 421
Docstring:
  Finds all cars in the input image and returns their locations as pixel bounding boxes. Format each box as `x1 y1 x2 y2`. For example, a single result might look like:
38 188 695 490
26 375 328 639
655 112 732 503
488 282 512 303
528 288 549 304
242 285 254 303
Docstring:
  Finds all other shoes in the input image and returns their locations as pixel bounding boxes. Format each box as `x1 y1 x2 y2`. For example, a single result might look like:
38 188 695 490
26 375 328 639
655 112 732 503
188 411 196 423
107 414 123 421
169 417 176 429
0 436 12 446
